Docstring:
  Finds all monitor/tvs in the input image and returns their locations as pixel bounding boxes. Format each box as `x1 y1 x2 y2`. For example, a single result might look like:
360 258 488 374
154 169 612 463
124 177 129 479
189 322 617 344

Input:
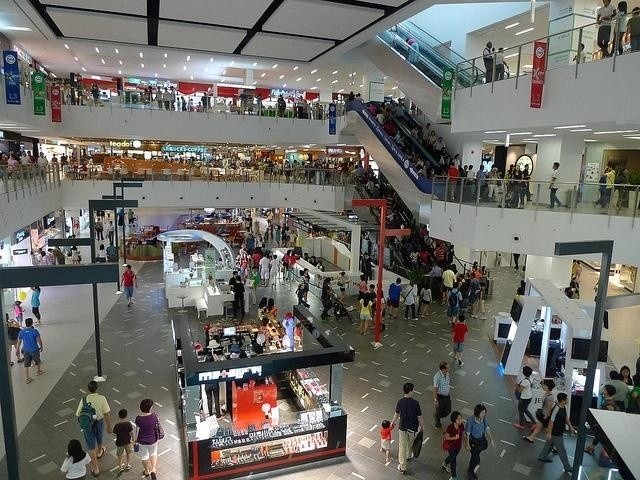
571 337 609 362
510 298 523 322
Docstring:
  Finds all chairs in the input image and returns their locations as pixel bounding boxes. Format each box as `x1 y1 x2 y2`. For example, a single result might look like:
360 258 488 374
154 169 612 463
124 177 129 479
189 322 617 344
62 162 266 182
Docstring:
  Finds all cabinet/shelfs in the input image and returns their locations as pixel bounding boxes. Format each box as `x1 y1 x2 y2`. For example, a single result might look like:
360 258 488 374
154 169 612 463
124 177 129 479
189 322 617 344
210 367 329 480
164 266 255 320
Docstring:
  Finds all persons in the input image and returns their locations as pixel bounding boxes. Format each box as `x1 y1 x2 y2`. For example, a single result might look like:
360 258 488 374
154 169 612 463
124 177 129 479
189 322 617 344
112 409 134 473
108 221 114 245
453 315 468 367
258 298 276 350
608 0 629 57
238 208 286 292
96 209 134 224
32 236 79 265
432 361 451 433
391 382 423 473
595 165 629 214
278 96 323 120
349 90 461 202
483 42 495 83
547 162 561 209
60 439 93 480
442 411 466 478
623 6 639 53
406 38 419 64
360 293 372 335
573 43 587 63
514 366 538 429
6 301 43 383
134 399 162 480
490 161 531 208
603 356 640 415
285 160 487 321
566 260 582 299
31 287 41 325
205 384 224 420
583 384 618 455
595 1 617 58
25 63 99 106
147 86 209 112
121 265 137 306
2 151 66 180
98 242 118 262
523 380 555 443
466 404 494 479
76 381 115 477
540 393 573 473
88 218 104 240
230 271 245 318
380 420 396 465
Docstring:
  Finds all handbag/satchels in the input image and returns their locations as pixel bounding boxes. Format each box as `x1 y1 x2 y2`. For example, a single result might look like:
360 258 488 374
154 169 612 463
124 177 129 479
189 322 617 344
515 384 521 400
387 297 392 306
536 408 544 422
442 432 451 450
134 444 139 452
470 437 488 452
155 421 164 439
7 327 21 340
337 281 343 286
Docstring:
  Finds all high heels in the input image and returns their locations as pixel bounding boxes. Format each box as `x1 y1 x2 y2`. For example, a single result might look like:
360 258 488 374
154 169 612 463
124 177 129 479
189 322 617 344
585 446 595 454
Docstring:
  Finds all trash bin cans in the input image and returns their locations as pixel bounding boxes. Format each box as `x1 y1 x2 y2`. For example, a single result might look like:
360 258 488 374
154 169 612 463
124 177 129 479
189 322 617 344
567 188 579 208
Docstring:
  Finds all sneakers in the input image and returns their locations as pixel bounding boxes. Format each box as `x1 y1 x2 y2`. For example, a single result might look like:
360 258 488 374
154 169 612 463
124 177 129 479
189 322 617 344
538 455 553 462
25 376 32 383
397 452 414 475
434 426 444 434
119 464 132 472
442 462 451 472
513 423 537 430
10 358 24 366
36 370 44 376
143 469 156 480
565 466 572 472
468 467 479 479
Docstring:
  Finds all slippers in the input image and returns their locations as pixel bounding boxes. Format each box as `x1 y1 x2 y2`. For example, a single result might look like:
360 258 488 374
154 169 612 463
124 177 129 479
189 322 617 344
523 435 534 443
97 446 106 458
91 467 99 477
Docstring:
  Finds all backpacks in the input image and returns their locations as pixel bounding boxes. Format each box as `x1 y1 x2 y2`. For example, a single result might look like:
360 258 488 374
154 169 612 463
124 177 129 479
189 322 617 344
449 290 460 306
576 267 581 275
609 171 623 184
78 396 98 429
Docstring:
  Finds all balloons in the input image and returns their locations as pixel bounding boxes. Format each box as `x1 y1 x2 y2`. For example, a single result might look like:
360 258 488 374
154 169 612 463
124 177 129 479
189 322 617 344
19 292 27 301
